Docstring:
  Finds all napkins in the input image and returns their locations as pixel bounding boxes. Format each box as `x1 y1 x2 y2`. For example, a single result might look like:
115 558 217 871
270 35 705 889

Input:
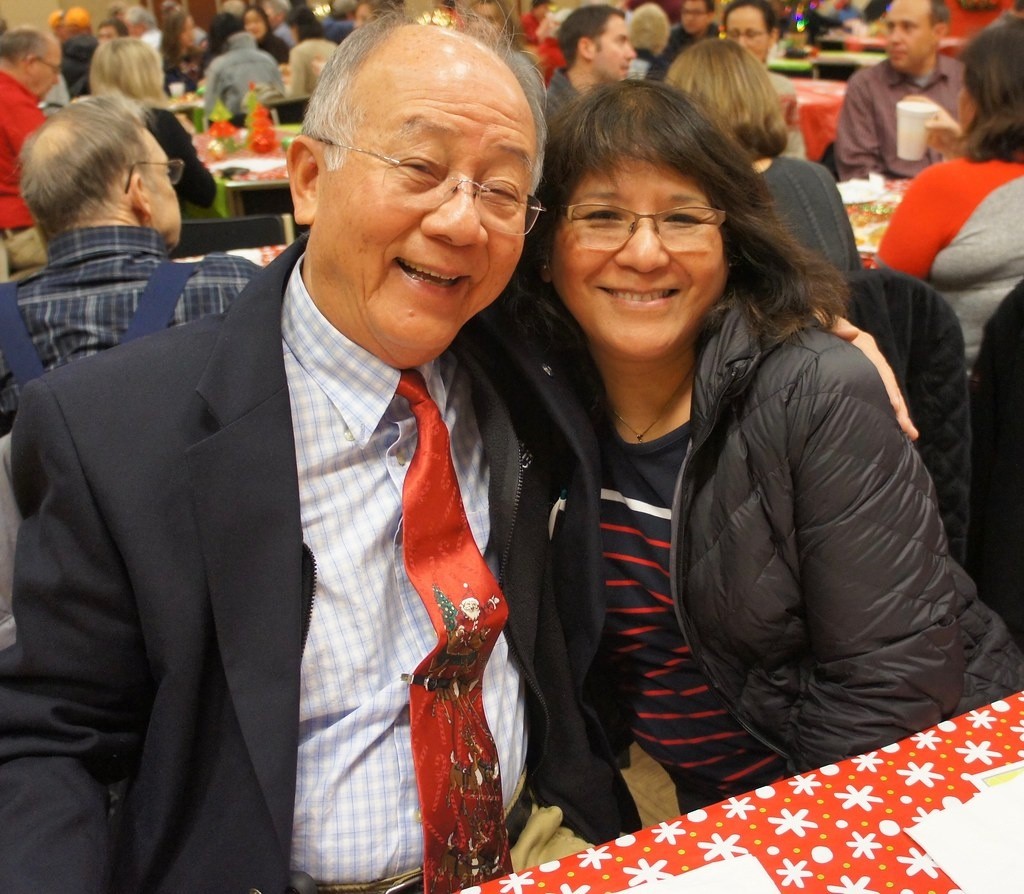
615 854 782 894
902 769 1024 894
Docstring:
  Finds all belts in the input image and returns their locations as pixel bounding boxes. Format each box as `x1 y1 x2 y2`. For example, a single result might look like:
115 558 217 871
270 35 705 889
386 781 533 894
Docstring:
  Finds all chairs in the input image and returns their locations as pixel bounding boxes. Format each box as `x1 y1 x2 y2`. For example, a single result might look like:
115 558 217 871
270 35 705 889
458 685 1024 894
970 276 1024 648
842 271 971 570
263 95 310 128
172 178 314 257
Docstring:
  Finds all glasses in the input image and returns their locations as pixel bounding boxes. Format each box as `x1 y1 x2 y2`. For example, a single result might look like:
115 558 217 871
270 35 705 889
318 137 549 236
125 159 185 194
40 58 61 75
552 204 726 252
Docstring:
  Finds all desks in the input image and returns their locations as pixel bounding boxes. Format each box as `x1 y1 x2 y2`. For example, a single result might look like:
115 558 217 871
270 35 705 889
839 179 911 269
796 77 849 163
192 121 304 216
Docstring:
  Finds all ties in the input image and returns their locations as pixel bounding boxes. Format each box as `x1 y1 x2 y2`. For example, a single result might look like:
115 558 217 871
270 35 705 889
397 368 515 894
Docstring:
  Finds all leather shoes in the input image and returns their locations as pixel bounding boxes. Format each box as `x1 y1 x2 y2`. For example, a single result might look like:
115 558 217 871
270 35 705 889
1 225 27 243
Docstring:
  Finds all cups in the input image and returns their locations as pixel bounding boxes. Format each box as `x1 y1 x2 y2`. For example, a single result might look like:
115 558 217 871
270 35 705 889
169 82 185 96
897 102 938 159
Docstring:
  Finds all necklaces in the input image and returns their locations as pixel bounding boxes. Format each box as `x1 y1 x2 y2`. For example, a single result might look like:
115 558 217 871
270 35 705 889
609 364 692 444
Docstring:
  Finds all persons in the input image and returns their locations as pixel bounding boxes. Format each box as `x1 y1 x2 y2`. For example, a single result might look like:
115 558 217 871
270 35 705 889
511 76 1024 873
470 0 1024 377
0 0 918 894
0 1 470 437
